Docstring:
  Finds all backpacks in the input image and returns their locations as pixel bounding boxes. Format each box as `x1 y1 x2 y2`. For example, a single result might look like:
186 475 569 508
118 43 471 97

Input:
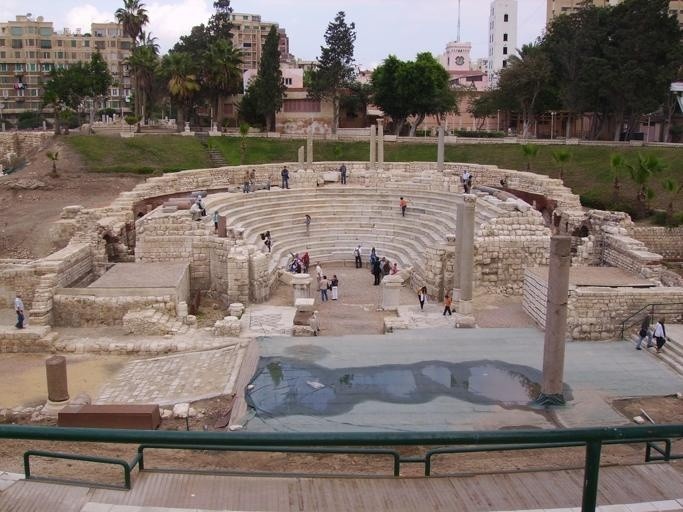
354 247 359 257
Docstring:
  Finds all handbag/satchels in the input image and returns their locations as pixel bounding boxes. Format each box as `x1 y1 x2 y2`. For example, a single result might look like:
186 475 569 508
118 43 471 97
639 328 647 338
652 330 657 338
327 282 330 290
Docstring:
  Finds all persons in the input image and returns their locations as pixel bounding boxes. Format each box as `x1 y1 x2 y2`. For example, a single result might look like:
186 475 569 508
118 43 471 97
213 211 219 234
281 166 289 189
305 214 311 236
289 250 338 301
264 231 271 253
190 195 207 221
340 163 346 185
443 295 452 316
243 170 250 193
462 170 471 193
15 291 25 329
352 245 399 286
400 197 407 216
636 310 655 350
653 317 667 353
250 169 256 192
418 286 427 310
